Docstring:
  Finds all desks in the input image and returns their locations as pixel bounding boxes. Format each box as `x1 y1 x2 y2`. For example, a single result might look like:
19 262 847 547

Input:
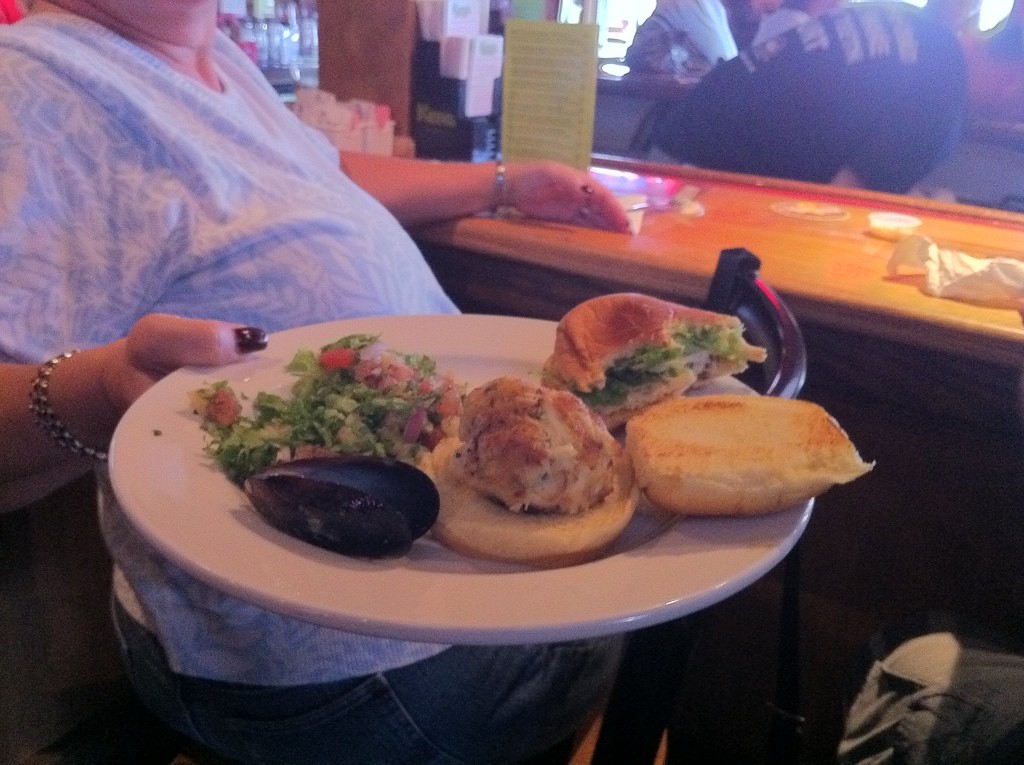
599 56 1023 159
417 154 1024 371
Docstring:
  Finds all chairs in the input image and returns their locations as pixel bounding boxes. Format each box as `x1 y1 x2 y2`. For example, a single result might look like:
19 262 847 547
564 251 806 764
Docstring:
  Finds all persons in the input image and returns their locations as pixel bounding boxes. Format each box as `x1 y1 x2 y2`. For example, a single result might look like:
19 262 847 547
626 1 1024 194
1 0 633 765
836 632 1023 765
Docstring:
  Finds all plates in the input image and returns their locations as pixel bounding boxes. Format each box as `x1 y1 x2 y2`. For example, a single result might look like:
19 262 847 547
105 312 816 646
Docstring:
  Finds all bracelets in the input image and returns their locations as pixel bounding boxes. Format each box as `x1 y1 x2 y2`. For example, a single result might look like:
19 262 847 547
492 161 505 212
26 349 107 462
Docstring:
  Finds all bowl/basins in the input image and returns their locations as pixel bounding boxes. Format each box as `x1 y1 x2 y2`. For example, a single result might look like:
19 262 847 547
869 213 921 240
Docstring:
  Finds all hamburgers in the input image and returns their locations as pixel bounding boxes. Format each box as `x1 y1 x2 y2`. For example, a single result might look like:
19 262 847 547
539 291 768 432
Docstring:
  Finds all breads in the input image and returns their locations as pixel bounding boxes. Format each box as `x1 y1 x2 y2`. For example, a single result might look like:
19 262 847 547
622 394 876 515
418 434 646 563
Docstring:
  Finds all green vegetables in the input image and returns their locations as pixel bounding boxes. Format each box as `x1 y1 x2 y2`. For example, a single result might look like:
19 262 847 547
187 330 466 484
